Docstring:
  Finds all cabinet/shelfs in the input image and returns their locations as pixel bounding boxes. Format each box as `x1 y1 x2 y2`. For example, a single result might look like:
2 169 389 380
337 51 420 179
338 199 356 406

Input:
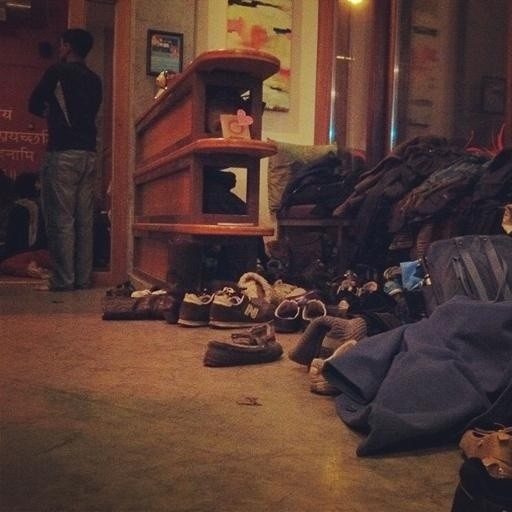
125 48 280 303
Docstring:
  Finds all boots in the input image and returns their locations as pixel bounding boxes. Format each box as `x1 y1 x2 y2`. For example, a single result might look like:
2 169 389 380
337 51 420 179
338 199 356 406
165 240 202 326
103 293 178 321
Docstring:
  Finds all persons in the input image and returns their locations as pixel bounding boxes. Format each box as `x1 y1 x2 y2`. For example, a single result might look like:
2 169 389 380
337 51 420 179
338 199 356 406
27 27 104 292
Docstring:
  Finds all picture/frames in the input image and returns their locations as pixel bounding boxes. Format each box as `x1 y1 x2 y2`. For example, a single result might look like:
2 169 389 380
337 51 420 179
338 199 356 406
145 28 184 77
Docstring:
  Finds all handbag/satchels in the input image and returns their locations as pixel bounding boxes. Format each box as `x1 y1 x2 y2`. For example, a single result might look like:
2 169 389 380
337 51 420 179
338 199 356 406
422 235 512 316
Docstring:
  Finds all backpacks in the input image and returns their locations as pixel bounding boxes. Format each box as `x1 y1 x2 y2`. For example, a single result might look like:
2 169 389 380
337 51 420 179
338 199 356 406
280 154 343 215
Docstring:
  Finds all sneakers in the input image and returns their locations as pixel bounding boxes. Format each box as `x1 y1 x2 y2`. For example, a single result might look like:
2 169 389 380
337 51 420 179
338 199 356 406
208 290 273 328
274 297 301 334
177 293 213 326
203 343 280 368
232 325 274 346
308 341 362 393
303 299 328 333
452 426 511 512
